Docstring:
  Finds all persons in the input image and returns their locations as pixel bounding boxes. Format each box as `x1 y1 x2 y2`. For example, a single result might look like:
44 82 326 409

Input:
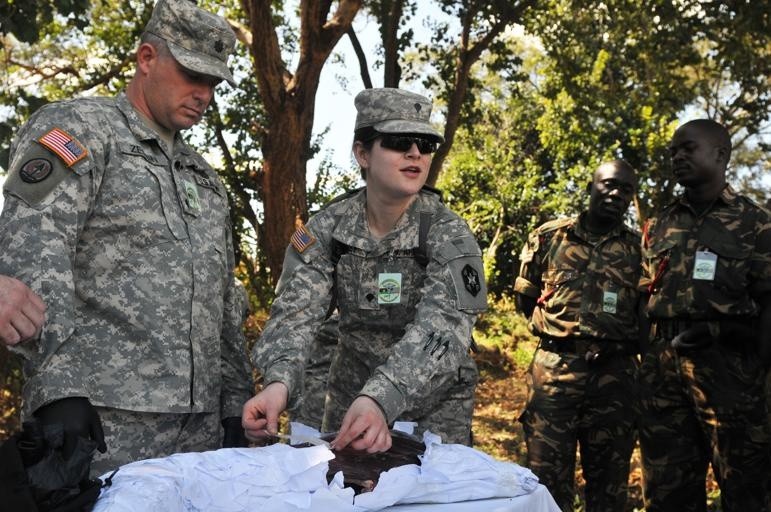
241 87 489 459
640 117 771 509
513 159 644 511
0 1 257 481
0 272 50 352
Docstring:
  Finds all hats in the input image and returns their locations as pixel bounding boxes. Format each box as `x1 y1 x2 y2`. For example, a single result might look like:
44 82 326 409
144 0 239 88
353 87 450 143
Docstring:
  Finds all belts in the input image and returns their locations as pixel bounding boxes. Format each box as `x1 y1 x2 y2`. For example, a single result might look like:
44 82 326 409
539 335 635 359
648 318 752 346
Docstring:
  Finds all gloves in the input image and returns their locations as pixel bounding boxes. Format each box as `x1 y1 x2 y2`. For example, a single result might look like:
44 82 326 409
219 417 247 448
37 399 109 455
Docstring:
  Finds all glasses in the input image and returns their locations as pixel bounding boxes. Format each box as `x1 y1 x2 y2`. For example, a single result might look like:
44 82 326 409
376 133 439 153
